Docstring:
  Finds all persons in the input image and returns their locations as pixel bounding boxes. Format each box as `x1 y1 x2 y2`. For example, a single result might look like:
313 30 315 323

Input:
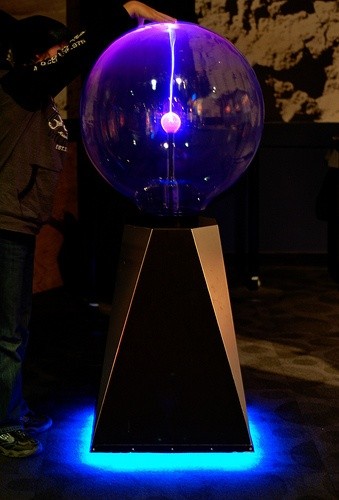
0 68 85 458
315 144 339 286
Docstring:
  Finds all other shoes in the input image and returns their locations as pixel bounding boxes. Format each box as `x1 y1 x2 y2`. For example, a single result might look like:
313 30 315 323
8 412 52 432
0 426 41 458
